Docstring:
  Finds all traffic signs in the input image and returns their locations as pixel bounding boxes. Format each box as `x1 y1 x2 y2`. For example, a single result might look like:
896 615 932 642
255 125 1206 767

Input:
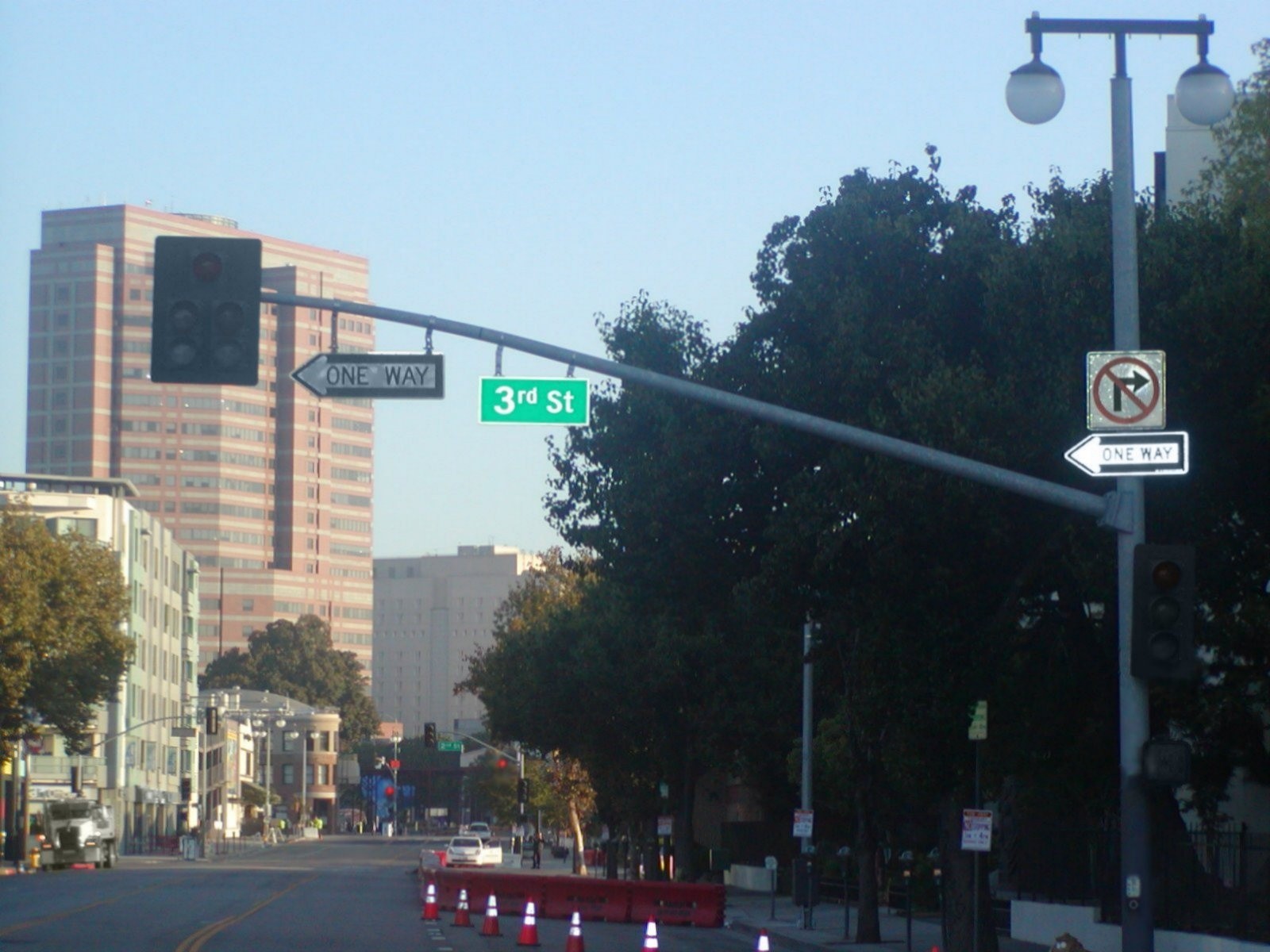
1063 430 1189 477
290 352 445 398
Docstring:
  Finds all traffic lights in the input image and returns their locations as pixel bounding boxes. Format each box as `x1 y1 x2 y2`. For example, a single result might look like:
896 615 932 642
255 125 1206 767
1129 537 1196 692
517 777 529 803
423 721 438 748
152 236 259 386
391 760 401 770
1140 736 1192 787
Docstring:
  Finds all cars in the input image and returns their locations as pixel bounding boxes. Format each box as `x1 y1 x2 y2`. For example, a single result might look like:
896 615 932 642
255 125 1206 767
443 834 503 868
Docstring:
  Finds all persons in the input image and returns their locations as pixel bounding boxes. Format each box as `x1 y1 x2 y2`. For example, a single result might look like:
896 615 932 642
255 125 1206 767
531 832 544 868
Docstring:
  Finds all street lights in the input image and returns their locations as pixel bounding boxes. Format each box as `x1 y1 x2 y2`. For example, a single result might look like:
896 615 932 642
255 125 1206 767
251 708 321 846
1006 9 1237 949
374 756 397 836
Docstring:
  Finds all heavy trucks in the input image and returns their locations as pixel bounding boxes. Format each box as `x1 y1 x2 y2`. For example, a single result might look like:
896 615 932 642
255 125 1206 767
27 795 116 869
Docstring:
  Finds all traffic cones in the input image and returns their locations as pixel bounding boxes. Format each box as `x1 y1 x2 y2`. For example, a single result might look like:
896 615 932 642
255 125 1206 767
476 887 504 937
565 906 584 952
517 896 543 947
753 927 771 952
639 914 660 952
419 879 442 922
448 883 474 928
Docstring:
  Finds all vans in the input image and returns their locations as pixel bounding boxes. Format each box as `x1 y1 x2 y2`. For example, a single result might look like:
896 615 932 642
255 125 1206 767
465 822 496 843
